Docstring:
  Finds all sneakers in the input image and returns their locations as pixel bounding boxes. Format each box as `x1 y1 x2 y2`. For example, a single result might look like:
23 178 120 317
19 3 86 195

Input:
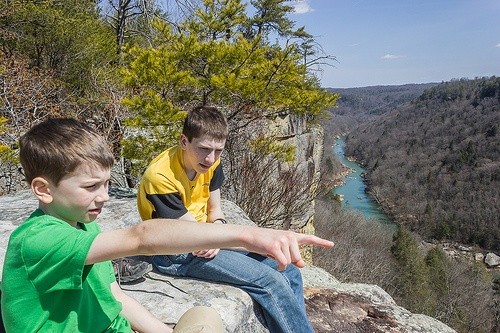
112 257 188 298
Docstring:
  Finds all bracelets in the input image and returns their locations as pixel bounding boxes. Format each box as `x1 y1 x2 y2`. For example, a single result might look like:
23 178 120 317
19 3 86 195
212 217 226 224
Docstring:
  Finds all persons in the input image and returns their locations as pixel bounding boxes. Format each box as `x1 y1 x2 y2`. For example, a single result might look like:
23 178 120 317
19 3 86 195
0 118 334 333
137 106 315 333
